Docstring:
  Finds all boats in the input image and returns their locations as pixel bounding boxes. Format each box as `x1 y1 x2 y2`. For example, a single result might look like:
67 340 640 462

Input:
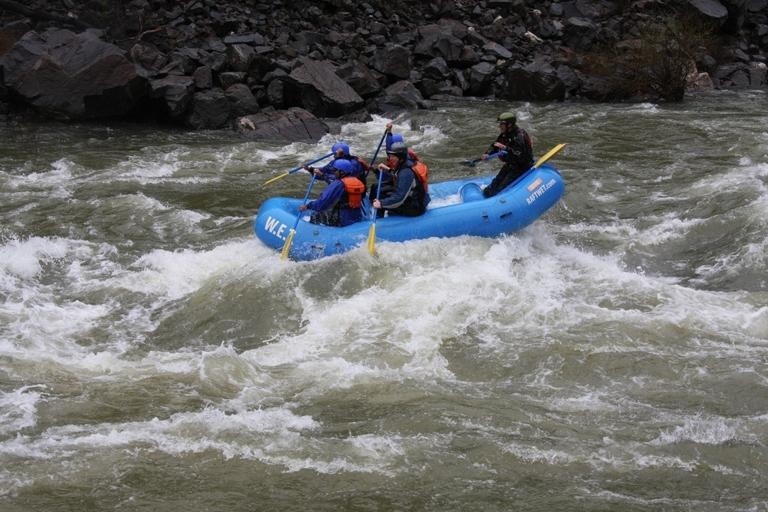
254 161 565 264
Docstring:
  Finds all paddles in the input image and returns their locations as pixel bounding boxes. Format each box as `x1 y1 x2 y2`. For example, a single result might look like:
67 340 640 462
458 151 508 167
486 143 567 196
263 153 334 186
367 168 383 256
279 170 317 263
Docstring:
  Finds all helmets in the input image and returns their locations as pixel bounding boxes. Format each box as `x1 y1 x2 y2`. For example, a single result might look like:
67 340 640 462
386 133 404 151
496 111 516 126
333 159 352 177
332 142 350 157
386 142 408 167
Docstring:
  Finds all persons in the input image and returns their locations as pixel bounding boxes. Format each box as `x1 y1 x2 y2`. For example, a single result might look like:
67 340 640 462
368 123 428 204
372 141 426 216
300 159 365 227
303 142 367 198
481 111 534 199
